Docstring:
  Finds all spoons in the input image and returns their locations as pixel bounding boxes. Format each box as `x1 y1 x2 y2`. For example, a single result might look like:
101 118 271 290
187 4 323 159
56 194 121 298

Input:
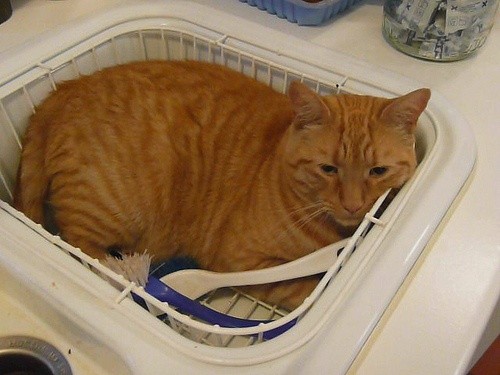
144 235 364 318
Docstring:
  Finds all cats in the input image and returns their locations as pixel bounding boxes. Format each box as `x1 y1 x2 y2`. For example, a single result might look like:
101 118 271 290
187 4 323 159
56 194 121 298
13 60 431 312
